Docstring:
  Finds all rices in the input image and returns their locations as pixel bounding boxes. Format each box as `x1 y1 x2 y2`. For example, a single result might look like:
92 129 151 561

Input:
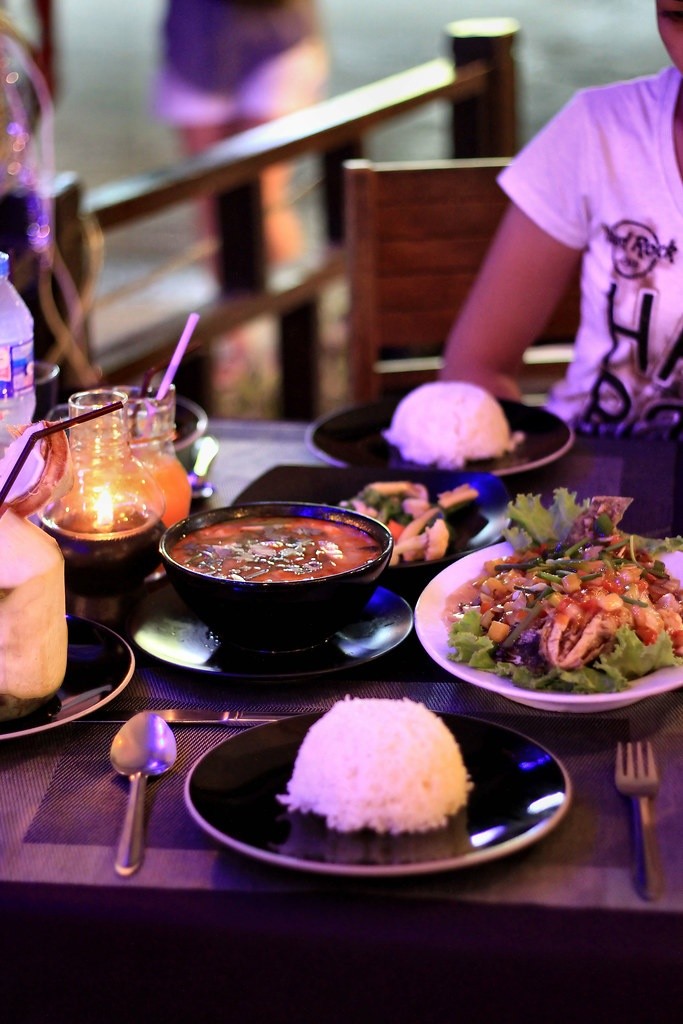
380 380 524 468
276 694 474 836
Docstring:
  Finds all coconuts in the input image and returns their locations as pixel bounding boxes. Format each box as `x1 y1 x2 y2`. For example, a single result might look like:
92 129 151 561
0 506 69 723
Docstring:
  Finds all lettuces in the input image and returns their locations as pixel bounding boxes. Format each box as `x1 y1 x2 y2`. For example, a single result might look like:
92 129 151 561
446 486 683 695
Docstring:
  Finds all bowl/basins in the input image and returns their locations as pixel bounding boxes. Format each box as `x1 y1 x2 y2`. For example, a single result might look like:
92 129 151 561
48 386 206 471
160 502 393 649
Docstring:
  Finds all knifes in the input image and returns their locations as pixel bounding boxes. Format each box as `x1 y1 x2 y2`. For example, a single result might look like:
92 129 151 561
69 709 306 725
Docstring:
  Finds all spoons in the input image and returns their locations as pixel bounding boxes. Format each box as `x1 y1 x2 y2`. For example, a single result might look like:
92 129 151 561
110 712 177 876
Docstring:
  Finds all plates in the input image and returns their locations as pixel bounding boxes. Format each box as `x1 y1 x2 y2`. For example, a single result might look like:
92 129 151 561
0 614 135 739
312 469 512 569
415 534 683 713
309 399 573 477
131 581 413 680
184 710 571 877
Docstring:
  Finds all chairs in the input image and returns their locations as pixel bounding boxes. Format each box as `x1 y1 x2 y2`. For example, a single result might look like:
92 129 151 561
346 158 586 405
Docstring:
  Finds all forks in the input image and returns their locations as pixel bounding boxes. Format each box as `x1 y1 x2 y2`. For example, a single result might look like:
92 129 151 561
615 741 664 901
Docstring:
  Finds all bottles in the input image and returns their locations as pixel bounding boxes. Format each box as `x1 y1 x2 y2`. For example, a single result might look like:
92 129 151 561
38 389 166 595
114 384 191 572
0 251 36 462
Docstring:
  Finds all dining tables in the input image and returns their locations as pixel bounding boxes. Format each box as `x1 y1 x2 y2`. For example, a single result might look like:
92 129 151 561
0 422 683 1024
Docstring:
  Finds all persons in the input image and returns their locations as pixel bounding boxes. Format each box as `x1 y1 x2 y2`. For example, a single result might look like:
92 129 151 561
154 0 323 270
436 1 683 444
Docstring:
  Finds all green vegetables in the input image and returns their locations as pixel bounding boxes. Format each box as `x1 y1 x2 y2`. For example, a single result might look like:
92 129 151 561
355 488 416 530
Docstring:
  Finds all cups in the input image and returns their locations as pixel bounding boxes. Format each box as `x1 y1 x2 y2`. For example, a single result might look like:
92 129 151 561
33 363 59 424
